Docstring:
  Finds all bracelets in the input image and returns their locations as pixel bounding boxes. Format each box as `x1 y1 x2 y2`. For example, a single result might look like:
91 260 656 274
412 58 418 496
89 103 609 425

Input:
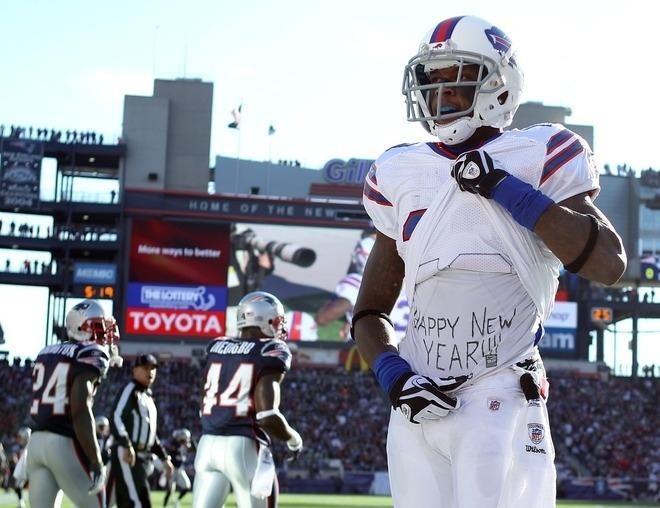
491 176 555 235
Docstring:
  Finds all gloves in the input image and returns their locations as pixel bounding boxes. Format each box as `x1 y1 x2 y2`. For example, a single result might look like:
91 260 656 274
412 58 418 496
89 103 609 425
287 428 303 452
388 371 469 422
88 464 106 496
450 149 511 198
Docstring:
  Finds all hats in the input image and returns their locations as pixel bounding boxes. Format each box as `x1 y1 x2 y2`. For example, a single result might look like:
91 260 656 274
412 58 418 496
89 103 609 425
132 353 157 369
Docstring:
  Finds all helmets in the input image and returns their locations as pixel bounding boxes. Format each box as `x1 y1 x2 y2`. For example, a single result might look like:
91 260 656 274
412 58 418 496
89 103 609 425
173 429 190 444
235 292 286 340
67 300 121 346
415 16 523 143
94 415 109 435
18 426 31 445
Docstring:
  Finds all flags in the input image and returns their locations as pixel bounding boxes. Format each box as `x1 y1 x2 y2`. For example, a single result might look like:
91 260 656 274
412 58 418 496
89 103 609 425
229 105 242 127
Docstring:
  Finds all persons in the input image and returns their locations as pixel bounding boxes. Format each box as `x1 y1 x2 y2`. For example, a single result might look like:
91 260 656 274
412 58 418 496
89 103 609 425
347 14 629 508
1 291 660 508
224 221 410 371
